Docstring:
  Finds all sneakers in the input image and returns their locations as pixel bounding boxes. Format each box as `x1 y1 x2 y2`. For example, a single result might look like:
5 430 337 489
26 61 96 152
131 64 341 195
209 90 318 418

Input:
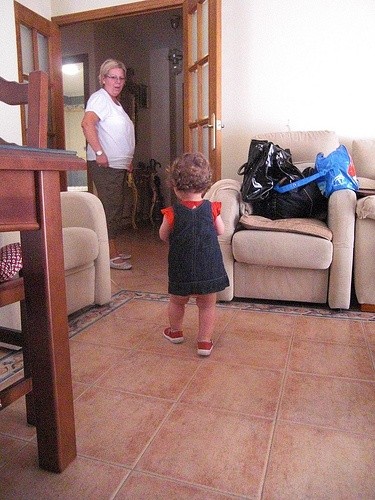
163 327 183 343
197 339 213 355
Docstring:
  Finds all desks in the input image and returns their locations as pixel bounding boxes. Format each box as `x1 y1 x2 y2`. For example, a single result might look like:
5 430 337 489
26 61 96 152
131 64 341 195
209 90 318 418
0 145 88 473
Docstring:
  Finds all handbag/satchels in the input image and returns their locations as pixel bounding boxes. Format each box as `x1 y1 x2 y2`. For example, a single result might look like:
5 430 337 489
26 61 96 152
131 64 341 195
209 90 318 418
238 140 359 220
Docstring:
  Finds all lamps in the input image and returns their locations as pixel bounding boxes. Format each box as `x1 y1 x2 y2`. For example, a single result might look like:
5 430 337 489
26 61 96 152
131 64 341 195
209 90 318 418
170 14 180 29
166 48 184 76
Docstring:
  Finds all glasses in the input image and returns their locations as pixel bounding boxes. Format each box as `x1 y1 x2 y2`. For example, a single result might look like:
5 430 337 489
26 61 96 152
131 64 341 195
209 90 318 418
104 75 127 82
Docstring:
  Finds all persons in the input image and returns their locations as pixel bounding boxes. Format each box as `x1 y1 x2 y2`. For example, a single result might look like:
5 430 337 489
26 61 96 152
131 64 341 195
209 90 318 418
81 58 136 270
159 152 230 356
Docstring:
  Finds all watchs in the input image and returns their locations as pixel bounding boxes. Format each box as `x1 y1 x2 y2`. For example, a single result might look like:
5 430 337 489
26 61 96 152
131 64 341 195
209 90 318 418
95 150 104 155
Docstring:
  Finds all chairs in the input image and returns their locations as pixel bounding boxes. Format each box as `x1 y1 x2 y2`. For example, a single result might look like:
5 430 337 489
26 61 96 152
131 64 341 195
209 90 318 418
0 70 49 427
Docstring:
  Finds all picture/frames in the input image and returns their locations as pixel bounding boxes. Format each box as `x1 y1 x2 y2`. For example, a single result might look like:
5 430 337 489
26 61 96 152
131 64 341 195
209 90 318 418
139 83 149 109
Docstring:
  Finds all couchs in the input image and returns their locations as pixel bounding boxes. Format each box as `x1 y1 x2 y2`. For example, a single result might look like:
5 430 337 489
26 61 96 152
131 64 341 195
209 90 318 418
203 131 375 312
0 191 112 332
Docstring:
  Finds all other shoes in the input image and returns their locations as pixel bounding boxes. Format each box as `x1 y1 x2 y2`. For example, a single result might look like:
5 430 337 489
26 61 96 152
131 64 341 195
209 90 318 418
119 254 131 259
110 257 132 269
